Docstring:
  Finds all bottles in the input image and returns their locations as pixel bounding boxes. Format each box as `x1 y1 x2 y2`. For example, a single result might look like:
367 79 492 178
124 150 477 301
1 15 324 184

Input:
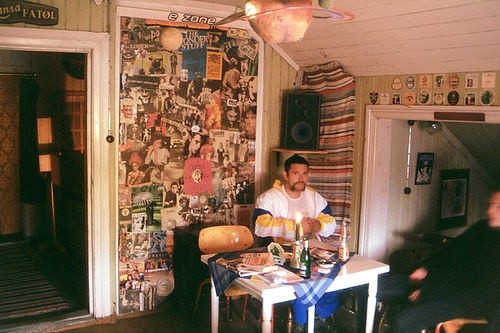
339 221 350 263
299 237 310 278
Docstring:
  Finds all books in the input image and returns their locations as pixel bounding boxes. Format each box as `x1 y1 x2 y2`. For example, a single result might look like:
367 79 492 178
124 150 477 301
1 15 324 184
217 250 305 287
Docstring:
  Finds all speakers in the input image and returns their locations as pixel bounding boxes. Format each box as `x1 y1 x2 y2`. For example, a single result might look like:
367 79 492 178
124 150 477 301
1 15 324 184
283 92 321 150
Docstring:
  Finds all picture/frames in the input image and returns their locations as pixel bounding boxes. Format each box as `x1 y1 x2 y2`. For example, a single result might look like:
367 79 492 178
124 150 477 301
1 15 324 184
414 152 434 186
438 168 470 231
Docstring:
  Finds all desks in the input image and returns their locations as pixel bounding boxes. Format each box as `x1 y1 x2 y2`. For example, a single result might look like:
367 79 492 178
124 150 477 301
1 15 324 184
201 238 390 333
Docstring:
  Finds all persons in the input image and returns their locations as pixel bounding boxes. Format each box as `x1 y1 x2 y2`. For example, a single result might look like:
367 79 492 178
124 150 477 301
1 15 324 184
390 190 500 333
120 17 258 315
252 155 337 247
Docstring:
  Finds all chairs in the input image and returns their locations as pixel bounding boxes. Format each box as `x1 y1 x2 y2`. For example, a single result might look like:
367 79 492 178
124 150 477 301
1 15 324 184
198 226 253 321
